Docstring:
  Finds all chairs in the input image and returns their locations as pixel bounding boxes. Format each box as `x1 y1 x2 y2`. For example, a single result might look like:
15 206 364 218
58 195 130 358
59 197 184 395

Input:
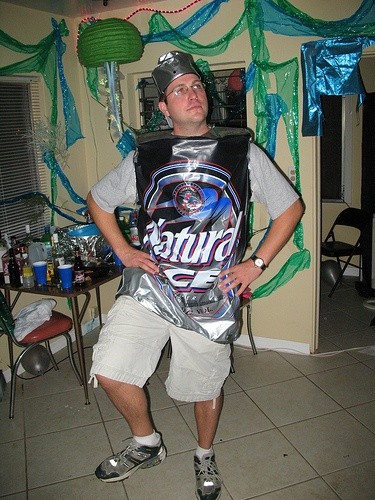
167 295 257 374
321 207 371 299
0 290 83 419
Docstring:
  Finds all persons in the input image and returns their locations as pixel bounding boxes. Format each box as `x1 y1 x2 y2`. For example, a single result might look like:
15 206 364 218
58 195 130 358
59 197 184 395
87 50 305 500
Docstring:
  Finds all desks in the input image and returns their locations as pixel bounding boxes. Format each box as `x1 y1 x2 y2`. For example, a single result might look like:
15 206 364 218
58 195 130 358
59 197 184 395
0 264 126 405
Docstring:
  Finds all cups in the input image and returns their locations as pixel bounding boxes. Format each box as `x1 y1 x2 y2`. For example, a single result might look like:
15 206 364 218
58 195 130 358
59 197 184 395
57 265 73 288
33 261 47 286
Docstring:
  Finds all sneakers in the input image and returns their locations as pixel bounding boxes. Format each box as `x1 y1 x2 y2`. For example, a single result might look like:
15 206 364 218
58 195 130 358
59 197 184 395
95 439 166 483
194 447 223 500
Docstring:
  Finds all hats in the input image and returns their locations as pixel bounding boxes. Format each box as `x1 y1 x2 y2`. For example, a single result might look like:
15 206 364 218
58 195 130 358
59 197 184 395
151 51 202 94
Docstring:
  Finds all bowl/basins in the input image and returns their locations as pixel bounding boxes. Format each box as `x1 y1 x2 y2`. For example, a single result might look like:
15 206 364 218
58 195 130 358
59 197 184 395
82 265 111 279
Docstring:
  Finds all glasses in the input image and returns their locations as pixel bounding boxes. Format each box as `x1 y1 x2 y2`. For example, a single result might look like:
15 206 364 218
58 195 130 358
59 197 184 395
166 84 204 97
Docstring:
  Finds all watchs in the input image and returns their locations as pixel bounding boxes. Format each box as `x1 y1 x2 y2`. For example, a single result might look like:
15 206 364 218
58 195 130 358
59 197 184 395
251 254 266 272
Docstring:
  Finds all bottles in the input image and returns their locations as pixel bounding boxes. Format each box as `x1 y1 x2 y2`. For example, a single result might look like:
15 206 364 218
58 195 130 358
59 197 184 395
24 224 35 253
8 248 21 287
20 253 34 288
112 212 141 269
42 227 54 263
74 245 85 287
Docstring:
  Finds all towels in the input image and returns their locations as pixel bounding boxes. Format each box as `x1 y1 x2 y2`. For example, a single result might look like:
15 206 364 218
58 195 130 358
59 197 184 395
12 299 58 343
